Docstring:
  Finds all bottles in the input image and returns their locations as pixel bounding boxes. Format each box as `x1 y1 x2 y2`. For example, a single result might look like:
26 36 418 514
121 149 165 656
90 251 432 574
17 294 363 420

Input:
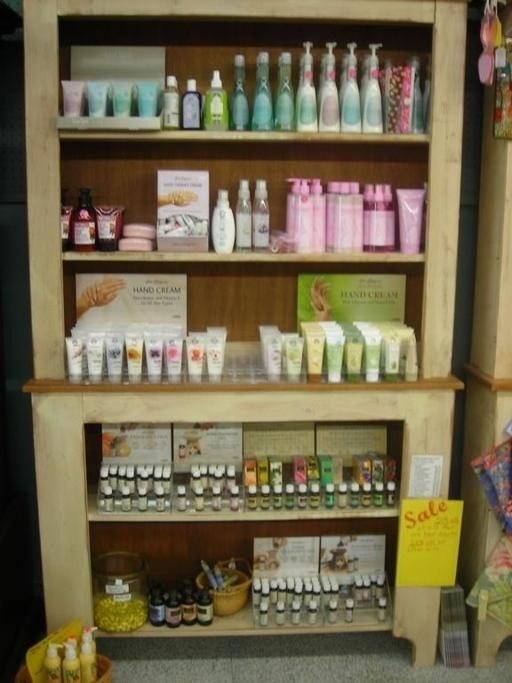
178 439 187 458
97 465 395 513
252 572 389 627
110 442 115 456
148 579 214 630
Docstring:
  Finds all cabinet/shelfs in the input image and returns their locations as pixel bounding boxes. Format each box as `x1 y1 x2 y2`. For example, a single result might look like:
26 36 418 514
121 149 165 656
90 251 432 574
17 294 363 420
466 0 512 668
23 1 468 670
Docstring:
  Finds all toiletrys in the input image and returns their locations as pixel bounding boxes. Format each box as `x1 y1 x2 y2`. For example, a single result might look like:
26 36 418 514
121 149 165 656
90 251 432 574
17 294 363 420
161 75 180 129
100 463 385 514
180 79 203 130
102 538 388 629
